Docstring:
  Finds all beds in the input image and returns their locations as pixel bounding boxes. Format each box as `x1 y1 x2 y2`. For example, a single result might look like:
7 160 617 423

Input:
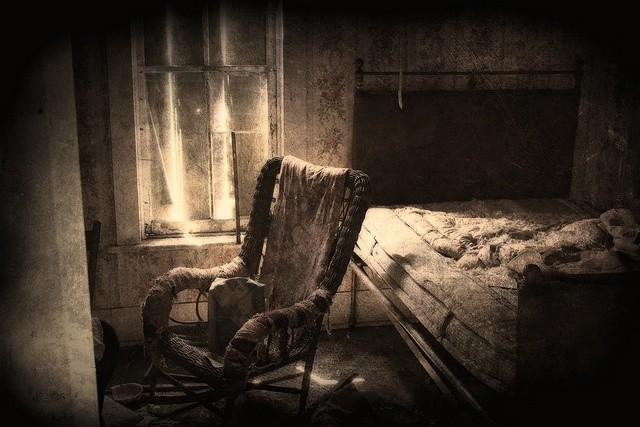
348 57 640 427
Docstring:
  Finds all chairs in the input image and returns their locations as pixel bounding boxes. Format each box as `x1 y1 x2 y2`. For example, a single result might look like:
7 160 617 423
141 155 371 427
